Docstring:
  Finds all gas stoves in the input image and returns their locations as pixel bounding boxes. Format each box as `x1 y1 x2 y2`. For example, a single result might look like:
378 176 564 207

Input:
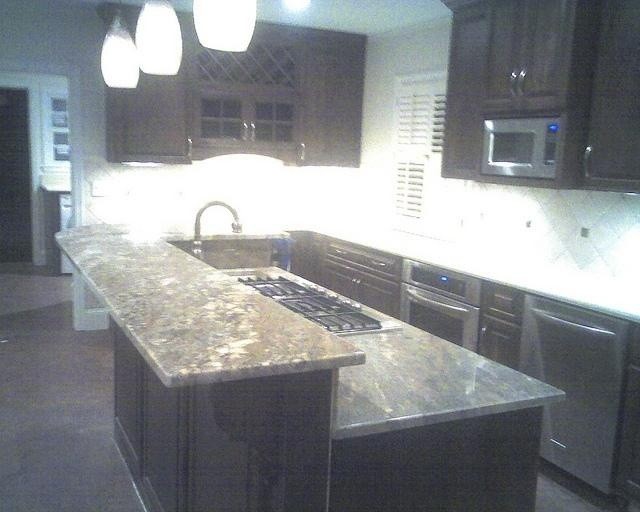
228 270 407 338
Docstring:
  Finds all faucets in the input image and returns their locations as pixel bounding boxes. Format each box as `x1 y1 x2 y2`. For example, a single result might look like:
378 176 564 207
194 201 243 240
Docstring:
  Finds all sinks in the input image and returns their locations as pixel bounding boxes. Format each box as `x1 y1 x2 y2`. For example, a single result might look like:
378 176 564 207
225 268 273 283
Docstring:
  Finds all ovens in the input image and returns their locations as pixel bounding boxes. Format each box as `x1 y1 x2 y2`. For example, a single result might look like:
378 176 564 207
398 259 484 359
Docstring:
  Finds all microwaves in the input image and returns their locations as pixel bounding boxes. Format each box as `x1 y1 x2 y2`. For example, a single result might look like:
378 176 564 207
479 116 563 179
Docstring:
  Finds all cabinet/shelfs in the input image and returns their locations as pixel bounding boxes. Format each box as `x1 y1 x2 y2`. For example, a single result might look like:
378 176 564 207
441 1 640 194
